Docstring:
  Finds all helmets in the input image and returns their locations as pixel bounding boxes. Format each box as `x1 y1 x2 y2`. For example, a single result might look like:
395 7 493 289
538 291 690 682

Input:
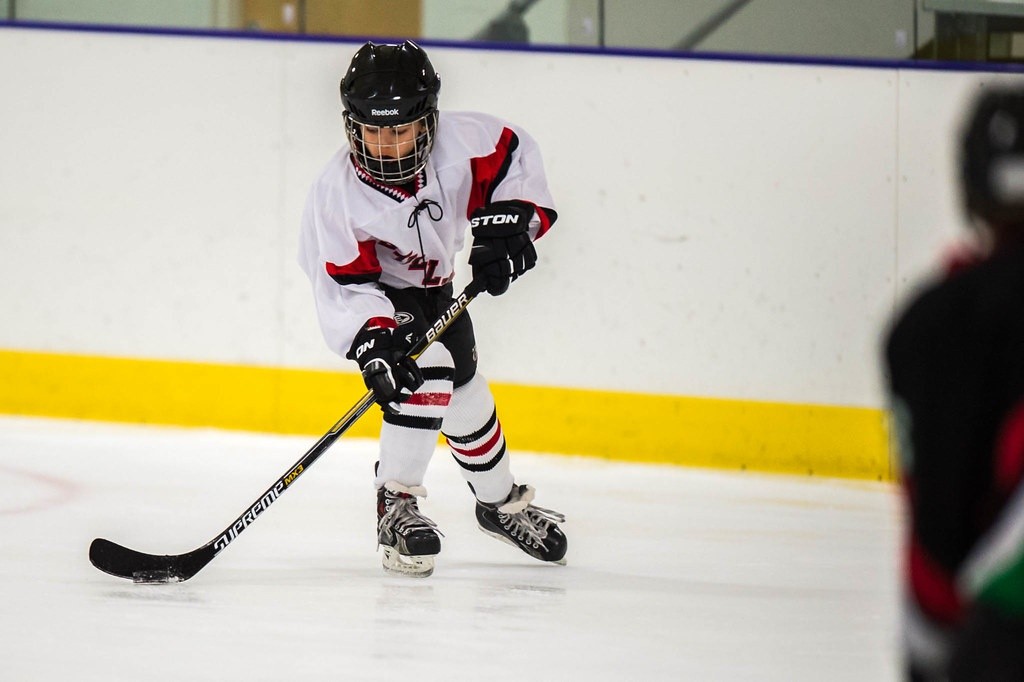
960 94 1024 231
340 39 441 186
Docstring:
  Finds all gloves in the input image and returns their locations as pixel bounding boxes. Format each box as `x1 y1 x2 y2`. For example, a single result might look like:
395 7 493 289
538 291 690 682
354 327 425 416
468 199 537 296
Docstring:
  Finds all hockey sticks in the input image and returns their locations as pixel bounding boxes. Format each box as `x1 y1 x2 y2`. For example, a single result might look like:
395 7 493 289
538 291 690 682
87 272 480 584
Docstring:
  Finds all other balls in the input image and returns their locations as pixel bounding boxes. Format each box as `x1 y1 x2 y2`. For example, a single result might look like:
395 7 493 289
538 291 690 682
131 569 171 584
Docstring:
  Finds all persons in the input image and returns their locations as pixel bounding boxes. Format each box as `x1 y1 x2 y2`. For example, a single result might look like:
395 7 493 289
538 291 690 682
299 39 570 578
870 73 1024 682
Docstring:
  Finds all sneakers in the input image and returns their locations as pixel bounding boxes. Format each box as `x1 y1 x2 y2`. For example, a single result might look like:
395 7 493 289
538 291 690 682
466 480 568 566
374 461 445 578
